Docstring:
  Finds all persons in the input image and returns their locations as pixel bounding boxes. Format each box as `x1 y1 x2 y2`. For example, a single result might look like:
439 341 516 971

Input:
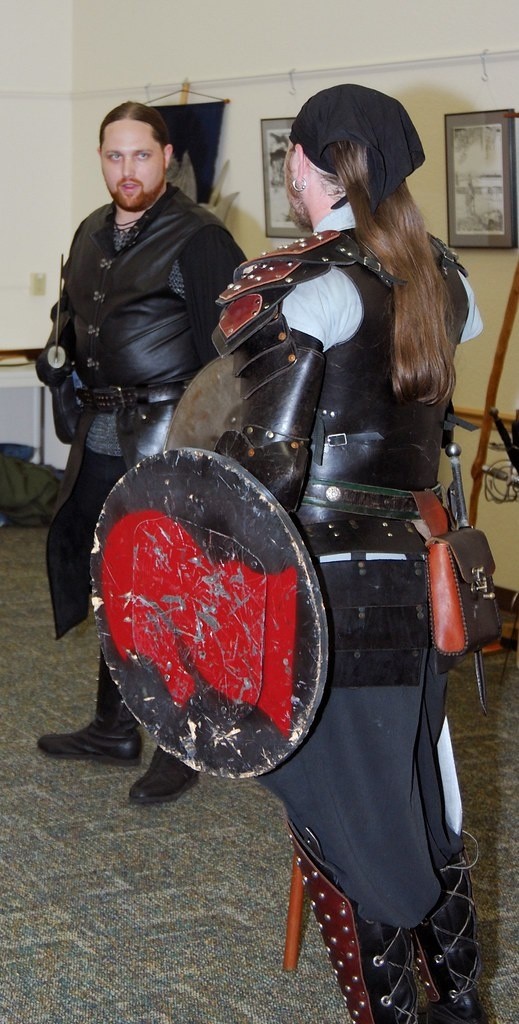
34 101 249 806
210 82 489 1024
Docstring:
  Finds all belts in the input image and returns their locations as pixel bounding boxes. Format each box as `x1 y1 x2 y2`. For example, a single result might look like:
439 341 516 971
300 477 445 521
76 379 194 413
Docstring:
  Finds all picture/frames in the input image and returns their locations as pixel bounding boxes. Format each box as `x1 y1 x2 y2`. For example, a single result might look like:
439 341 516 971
261 117 313 238
444 109 516 249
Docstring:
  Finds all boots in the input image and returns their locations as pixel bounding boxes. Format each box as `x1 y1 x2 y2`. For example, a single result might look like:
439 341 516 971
37 650 142 767
128 746 200 804
285 819 420 1024
409 828 490 1024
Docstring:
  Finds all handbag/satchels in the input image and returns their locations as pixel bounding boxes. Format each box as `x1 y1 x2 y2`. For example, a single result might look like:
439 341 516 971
424 527 502 658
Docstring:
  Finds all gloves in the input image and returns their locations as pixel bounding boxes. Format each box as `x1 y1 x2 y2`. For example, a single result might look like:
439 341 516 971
36 339 74 388
